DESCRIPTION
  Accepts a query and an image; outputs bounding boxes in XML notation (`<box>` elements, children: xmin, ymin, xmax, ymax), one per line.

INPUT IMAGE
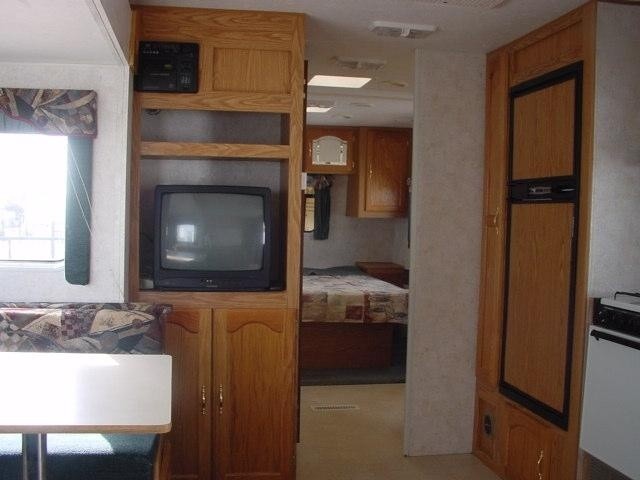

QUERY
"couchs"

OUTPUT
<box><xmin>0</xmin><ymin>304</ymin><xmax>173</xmax><ymax>479</ymax></box>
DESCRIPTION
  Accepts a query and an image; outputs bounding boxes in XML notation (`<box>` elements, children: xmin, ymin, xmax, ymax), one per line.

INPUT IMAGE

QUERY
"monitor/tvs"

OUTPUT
<box><xmin>153</xmin><ymin>185</ymin><xmax>272</xmax><ymax>292</ymax></box>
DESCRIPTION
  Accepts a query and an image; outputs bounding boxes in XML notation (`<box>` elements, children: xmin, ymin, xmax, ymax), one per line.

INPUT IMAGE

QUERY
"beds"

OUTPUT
<box><xmin>299</xmin><ymin>268</ymin><xmax>410</xmax><ymax>370</ymax></box>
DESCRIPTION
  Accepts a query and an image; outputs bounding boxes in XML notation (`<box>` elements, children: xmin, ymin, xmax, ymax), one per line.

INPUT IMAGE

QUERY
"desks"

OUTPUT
<box><xmin>0</xmin><ymin>351</ymin><xmax>173</xmax><ymax>479</ymax></box>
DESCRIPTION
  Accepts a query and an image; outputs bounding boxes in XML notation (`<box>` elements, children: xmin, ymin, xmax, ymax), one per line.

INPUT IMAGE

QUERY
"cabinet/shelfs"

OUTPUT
<box><xmin>344</xmin><ymin>128</ymin><xmax>415</xmax><ymax>220</ymax></box>
<box><xmin>303</xmin><ymin>123</ymin><xmax>357</xmax><ymax>173</ymax></box>
<box><xmin>469</xmin><ymin>0</ymin><xmax>598</xmax><ymax>480</ymax></box>
<box><xmin>129</xmin><ymin>7</ymin><xmax>306</xmax><ymax>480</ymax></box>
